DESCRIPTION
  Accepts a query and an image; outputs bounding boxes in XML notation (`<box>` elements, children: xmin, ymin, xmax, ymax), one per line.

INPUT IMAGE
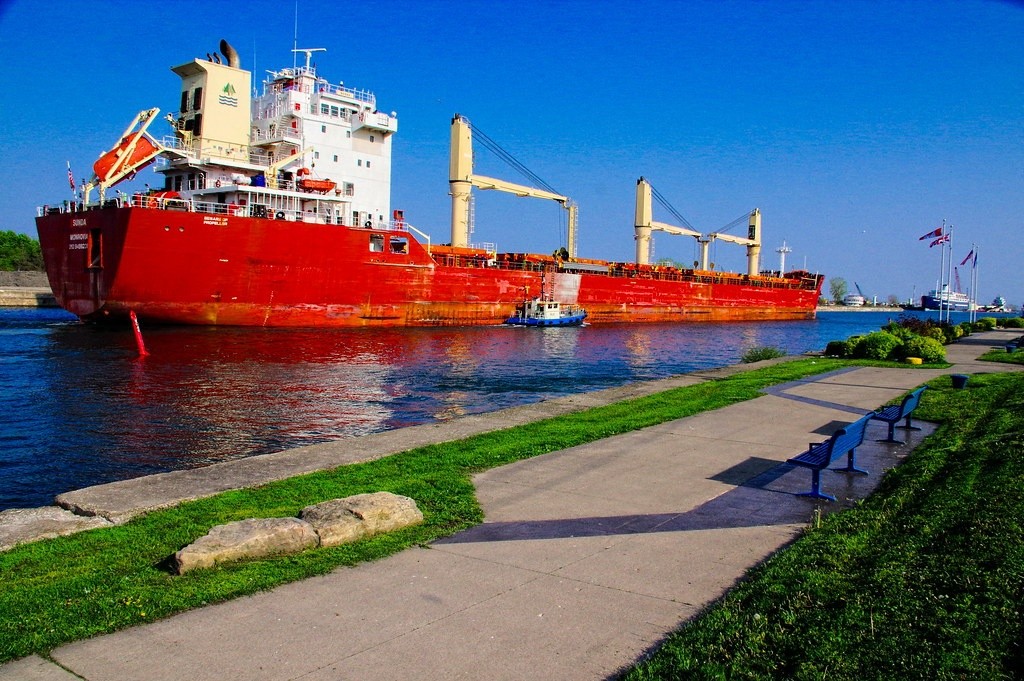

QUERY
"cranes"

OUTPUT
<box><xmin>953</xmin><ymin>267</ymin><xmax>961</xmax><ymax>293</ymax></box>
<box><xmin>854</xmin><ymin>281</ymin><xmax>861</xmax><ymax>296</ymax></box>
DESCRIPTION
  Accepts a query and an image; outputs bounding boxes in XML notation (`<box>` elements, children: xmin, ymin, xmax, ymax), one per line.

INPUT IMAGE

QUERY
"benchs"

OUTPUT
<box><xmin>869</xmin><ymin>385</ymin><xmax>926</xmax><ymax>446</ymax></box>
<box><xmin>789</xmin><ymin>414</ymin><xmax>873</xmax><ymax>501</ymax></box>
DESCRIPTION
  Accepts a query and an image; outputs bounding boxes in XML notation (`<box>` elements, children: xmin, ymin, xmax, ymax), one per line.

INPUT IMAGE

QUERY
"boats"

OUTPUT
<box><xmin>300</xmin><ymin>178</ymin><xmax>336</xmax><ymax>191</ymax></box>
<box><xmin>502</xmin><ymin>273</ymin><xmax>588</xmax><ymax>328</ymax></box>
<box><xmin>921</xmin><ymin>280</ymin><xmax>969</xmax><ymax>311</ymax></box>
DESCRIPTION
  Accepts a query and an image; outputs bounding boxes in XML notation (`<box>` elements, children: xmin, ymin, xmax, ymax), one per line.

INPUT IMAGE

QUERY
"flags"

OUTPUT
<box><xmin>972</xmin><ymin>251</ymin><xmax>977</xmax><ymax>267</ymax></box>
<box><xmin>68</xmin><ymin>158</ymin><xmax>75</xmax><ymax>189</ymax></box>
<box><xmin>929</xmin><ymin>233</ymin><xmax>950</xmax><ymax>248</ymax></box>
<box><xmin>960</xmin><ymin>249</ymin><xmax>973</xmax><ymax>265</ymax></box>
<box><xmin>919</xmin><ymin>227</ymin><xmax>943</xmax><ymax>240</ymax></box>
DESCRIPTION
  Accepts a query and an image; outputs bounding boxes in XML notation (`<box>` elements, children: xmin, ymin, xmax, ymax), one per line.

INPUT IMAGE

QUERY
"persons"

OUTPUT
<box><xmin>228</xmin><ymin>200</ymin><xmax>240</xmax><ymax>215</ymax></box>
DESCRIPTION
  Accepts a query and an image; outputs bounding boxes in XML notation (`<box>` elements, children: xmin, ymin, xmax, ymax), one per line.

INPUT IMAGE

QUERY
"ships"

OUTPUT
<box><xmin>34</xmin><ymin>0</ymin><xmax>826</xmax><ymax>356</ymax></box>
